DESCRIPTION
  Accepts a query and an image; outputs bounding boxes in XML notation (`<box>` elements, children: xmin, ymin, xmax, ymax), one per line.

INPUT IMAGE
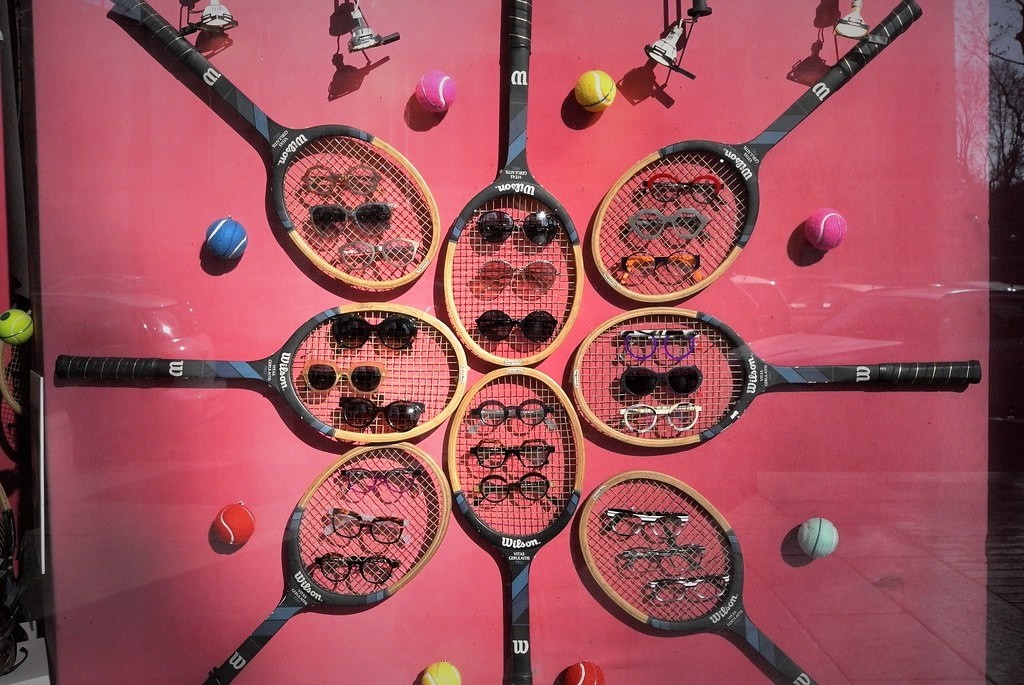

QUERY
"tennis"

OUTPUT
<box><xmin>802</xmin><ymin>206</ymin><xmax>848</xmax><ymax>251</ymax></box>
<box><xmin>0</xmin><ymin>309</ymin><xmax>35</xmax><ymax>346</ymax></box>
<box><xmin>214</xmin><ymin>502</ymin><xmax>256</xmax><ymax>546</ymax></box>
<box><xmin>206</xmin><ymin>218</ymin><xmax>248</xmax><ymax>261</ymax></box>
<box><xmin>415</xmin><ymin>70</ymin><xmax>456</xmax><ymax>113</ymax></box>
<box><xmin>574</xmin><ymin>68</ymin><xmax>618</xmax><ymax>112</ymax></box>
<box><xmin>423</xmin><ymin>661</ymin><xmax>463</xmax><ymax>685</ymax></box>
<box><xmin>798</xmin><ymin>516</ymin><xmax>840</xmax><ymax>558</ymax></box>
<box><xmin>563</xmin><ymin>659</ymin><xmax>606</xmax><ymax>685</ymax></box>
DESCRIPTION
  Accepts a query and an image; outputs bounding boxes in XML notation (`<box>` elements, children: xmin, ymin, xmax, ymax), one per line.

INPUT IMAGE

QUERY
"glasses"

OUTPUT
<box><xmin>315</xmin><ymin>552</ymin><xmax>402</xmax><ymax>584</ymax></box>
<box><xmin>471</xmin><ymin>400</ymin><xmax>556</xmax><ymax>432</ymax></box>
<box><xmin>642</xmin><ymin>171</ymin><xmax>726</xmax><ymax>203</ymax></box>
<box><xmin>469</xmin><ymin>436</ymin><xmax>556</xmax><ymax>469</ymax></box>
<box><xmin>302</xmin><ymin>162</ymin><xmax>383</xmax><ymax>196</ymax></box>
<box><xmin>476</xmin><ymin>211</ymin><xmax>561</xmax><ymax>245</ymax></box>
<box><xmin>302</xmin><ymin>360</ymin><xmax>387</xmax><ymax>394</ymax></box>
<box><xmin>327</xmin><ymin>508</ymin><xmax>408</xmax><ymax>544</ymax></box>
<box><xmin>622</xmin><ymin>544</ymin><xmax>706</xmax><ymax>573</ymax></box>
<box><xmin>335</xmin><ymin>238</ymin><xmax>426</xmax><ymax>270</ymax></box>
<box><xmin>474</xmin><ymin>472</ymin><xmax>553</xmax><ymax>512</ymax></box>
<box><xmin>647</xmin><ymin>573</ymin><xmax>730</xmax><ymax>605</ymax></box>
<box><xmin>340</xmin><ymin>469</ymin><xmax>421</xmax><ymax>495</ymax></box>
<box><xmin>308</xmin><ymin>203</ymin><xmax>397</xmax><ymax>236</ymax></box>
<box><xmin>621</xmin><ymin>208</ymin><xmax>712</xmax><ymax>243</ymax></box>
<box><xmin>331</xmin><ymin>316</ymin><xmax>419</xmax><ymax>353</ymax></box>
<box><xmin>475</xmin><ymin>311</ymin><xmax>559</xmax><ymax>343</ymax></box>
<box><xmin>620</xmin><ymin>250</ymin><xmax>702</xmax><ymax>287</ymax></box>
<box><xmin>604</xmin><ymin>507</ymin><xmax>689</xmax><ymax>546</ymax></box>
<box><xmin>617</xmin><ymin>329</ymin><xmax>701</xmax><ymax>364</ymax></box>
<box><xmin>476</xmin><ymin>259</ymin><xmax>560</xmax><ymax>293</ymax></box>
<box><xmin>616</xmin><ymin>364</ymin><xmax>704</xmax><ymax>403</ymax></box>
<box><xmin>619</xmin><ymin>404</ymin><xmax>702</xmax><ymax>433</ymax></box>
<box><xmin>338</xmin><ymin>398</ymin><xmax>426</xmax><ymax>432</ymax></box>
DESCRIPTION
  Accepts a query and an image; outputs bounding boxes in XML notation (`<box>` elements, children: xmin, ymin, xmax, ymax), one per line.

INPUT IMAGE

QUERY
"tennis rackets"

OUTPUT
<box><xmin>571</xmin><ymin>307</ymin><xmax>983</xmax><ymax>448</ymax></box>
<box><xmin>106</xmin><ymin>0</ymin><xmax>443</xmax><ymax>292</ymax></box>
<box><xmin>51</xmin><ymin>301</ymin><xmax>468</xmax><ymax>444</ymax></box>
<box><xmin>589</xmin><ymin>0</ymin><xmax>925</xmax><ymax>303</ymax></box>
<box><xmin>576</xmin><ymin>469</ymin><xmax>821</xmax><ymax>685</ymax></box>
<box><xmin>200</xmin><ymin>443</ymin><xmax>451</xmax><ymax>684</ymax></box>
<box><xmin>447</xmin><ymin>366</ymin><xmax>589</xmax><ymax>685</ymax></box>
<box><xmin>443</xmin><ymin>1</ymin><xmax>586</xmax><ymax>368</ymax></box>
